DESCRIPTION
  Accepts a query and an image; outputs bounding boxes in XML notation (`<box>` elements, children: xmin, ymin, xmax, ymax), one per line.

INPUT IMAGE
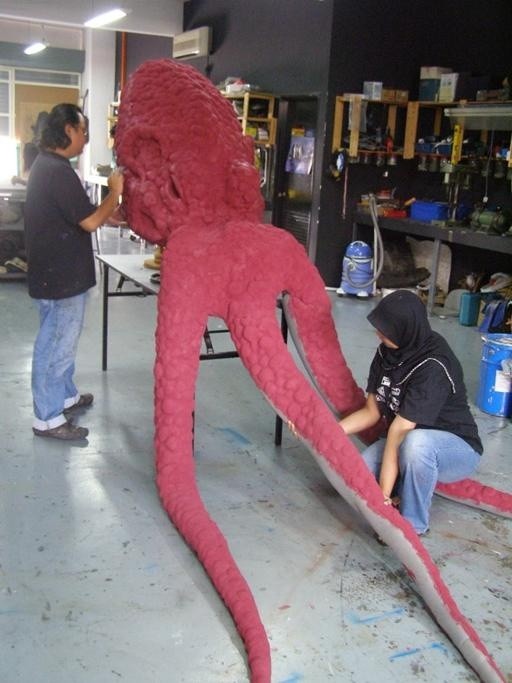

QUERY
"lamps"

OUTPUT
<box><xmin>23</xmin><ymin>22</ymin><xmax>49</xmax><ymax>56</ymax></box>
<box><xmin>83</xmin><ymin>0</ymin><xmax>127</xmax><ymax>29</ymax></box>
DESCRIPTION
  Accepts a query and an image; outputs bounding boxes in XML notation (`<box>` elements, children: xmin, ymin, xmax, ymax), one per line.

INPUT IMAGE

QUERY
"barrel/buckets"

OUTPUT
<box><xmin>459</xmin><ymin>292</ymin><xmax>478</xmax><ymax>327</ymax></box>
<box><xmin>478</xmin><ymin>334</ymin><xmax>512</xmax><ymax>418</ymax></box>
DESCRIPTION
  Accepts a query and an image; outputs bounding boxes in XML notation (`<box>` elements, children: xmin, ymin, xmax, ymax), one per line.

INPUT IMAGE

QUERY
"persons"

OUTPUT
<box><xmin>11</xmin><ymin>141</ymin><xmax>39</xmax><ymax>187</ymax></box>
<box><xmin>287</xmin><ymin>289</ymin><xmax>486</xmax><ymax>548</ymax></box>
<box><xmin>22</xmin><ymin>102</ymin><xmax>125</xmax><ymax>442</ymax></box>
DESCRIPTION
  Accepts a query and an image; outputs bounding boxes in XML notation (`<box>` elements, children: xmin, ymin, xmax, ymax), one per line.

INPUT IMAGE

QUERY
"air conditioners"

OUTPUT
<box><xmin>172</xmin><ymin>25</ymin><xmax>212</xmax><ymax>62</ymax></box>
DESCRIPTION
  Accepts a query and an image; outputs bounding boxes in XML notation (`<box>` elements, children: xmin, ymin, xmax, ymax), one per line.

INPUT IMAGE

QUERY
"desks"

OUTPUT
<box><xmin>347</xmin><ymin>210</ymin><xmax>512</xmax><ymax>319</ymax></box>
<box><xmin>93</xmin><ymin>252</ymin><xmax>289</xmax><ymax>447</ymax></box>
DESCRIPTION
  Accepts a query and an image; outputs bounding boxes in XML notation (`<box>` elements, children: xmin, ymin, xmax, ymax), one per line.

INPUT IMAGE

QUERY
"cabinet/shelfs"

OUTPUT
<box><xmin>223</xmin><ymin>91</ymin><xmax>277</xmax><ymax>147</ymax></box>
<box><xmin>332</xmin><ymin>94</ymin><xmax>511</xmax><ymax>169</ymax></box>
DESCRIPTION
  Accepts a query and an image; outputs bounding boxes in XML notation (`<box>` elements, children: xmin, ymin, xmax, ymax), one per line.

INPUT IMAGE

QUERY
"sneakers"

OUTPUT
<box><xmin>63</xmin><ymin>392</ymin><xmax>93</xmax><ymax>414</ymax></box>
<box><xmin>32</xmin><ymin>421</ymin><xmax>89</xmax><ymax>440</ymax></box>
